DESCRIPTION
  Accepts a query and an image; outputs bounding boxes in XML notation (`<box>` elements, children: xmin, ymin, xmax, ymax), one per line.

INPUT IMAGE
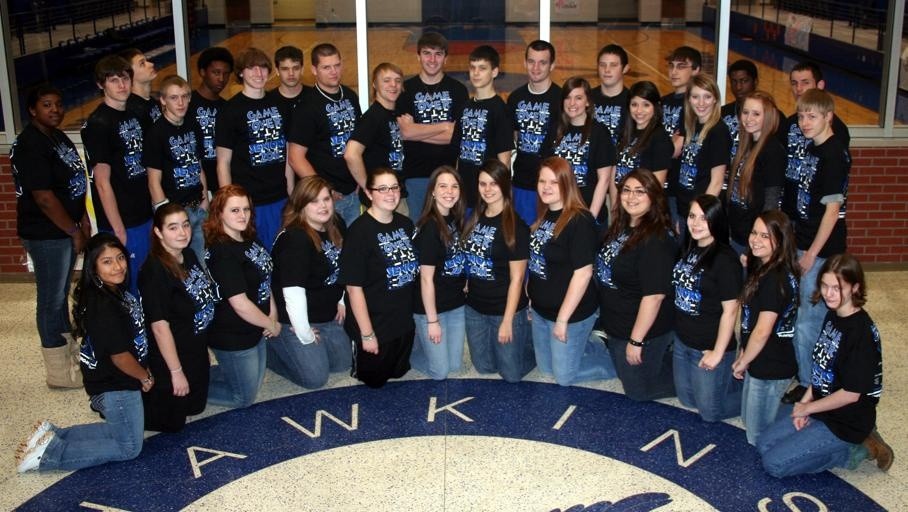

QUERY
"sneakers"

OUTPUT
<box><xmin>15</xmin><ymin>419</ymin><xmax>53</xmax><ymax>465</ymax></box>
<box><xmin>18</xmin><ymin>431</ymin><xmax>56</xmax><ymax>475</ymax></box>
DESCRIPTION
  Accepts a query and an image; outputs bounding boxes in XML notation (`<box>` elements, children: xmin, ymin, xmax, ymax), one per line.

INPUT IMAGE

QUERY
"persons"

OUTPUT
<box><xmin>81</xmin><ymin>32</ymin><xmax>854</xmax><ymax>451</ymax></box>
<box><xmin>755</xmin><ymin>254</ymin><xmax>895</xmax><ymax>479</ymax></box>
<box><xmin>13</xmin><ymin>230</ymin><xmax>156</xmax><ymax>474</ymax></box>
<box><xmin>11</xmin><ymin>81</ymin><xmax>93</xmax><ymax>391</ymax></box>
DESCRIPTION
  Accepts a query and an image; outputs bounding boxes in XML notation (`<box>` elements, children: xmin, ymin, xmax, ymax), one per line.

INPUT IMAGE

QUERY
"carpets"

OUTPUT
<box><xmin>406</xmin><ymin>26</ymin><xmax>524</xmax><ymax>43</ymax></box>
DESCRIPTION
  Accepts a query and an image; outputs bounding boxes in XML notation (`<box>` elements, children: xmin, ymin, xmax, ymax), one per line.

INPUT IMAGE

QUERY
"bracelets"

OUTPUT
<box><xmin>68</xmin><ymin>221</ymin><xmax>80</xmax><ymax>236</ymax></box>
<box><xmin>140</xmin><ymin>371</ymin><xmax>152</xmax><ymax>386</ymax></box>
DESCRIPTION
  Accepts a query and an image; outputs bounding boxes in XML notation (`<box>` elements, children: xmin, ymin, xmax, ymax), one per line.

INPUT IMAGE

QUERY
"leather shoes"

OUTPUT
<box><xmin>780</xmin><ymin>385</ymin><xmax>808</xmax><ymax>405</ymax></box>
<box><xmin>863</xmin><ymin>430</ymin><xmax>894</xmax><ymax>471</ymax></box>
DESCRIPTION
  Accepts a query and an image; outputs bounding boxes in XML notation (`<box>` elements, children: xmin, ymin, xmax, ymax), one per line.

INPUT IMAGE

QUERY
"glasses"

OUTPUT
<box><xmin>369</xmin><ymin>184</ymin><xmax>401</xmax><ymax>194</ymax></box>
<box><xmin>620</xmin><ymin>187</ymin><xmax>648</xmax><ymax>197</ymax></box>
<box><xmin>665</xmin><ymin>62</ymin><xmax>692</xmax><ymax>69</ymax></box>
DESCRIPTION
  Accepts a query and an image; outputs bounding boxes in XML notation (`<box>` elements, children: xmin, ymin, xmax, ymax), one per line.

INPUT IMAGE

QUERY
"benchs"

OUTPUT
<box><xmin>143</xmin><ymin>44</ymin><xmax>175</xmax><ymax>64</ymax></box>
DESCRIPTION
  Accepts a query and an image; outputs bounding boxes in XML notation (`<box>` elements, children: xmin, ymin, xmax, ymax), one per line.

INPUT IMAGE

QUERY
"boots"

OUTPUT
<box><xmin>62</xmin><ymin>333</ymin><xmax>82</xmax><ymax>365</ymax></box>
<box><xmin>42</xmin><ymin>346</ymin><xmax>84</xmax><ymax>391</ymax></box>
